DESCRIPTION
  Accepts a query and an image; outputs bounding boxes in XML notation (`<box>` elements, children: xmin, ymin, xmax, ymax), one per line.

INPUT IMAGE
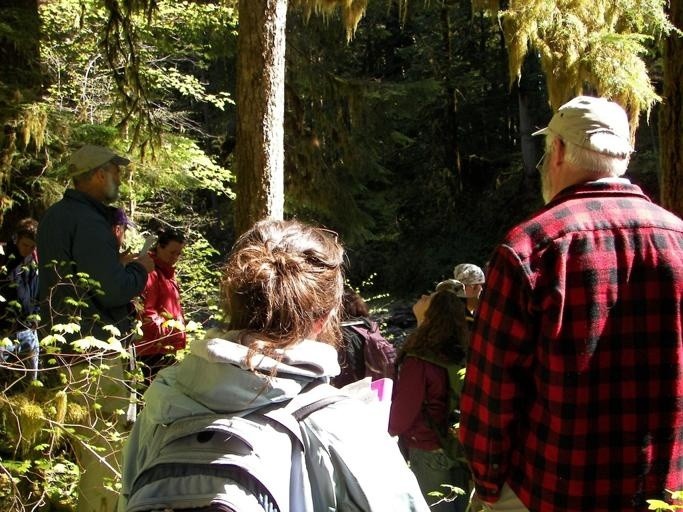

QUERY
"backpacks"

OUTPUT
<box><xmin>125</xmin><ymin>387</ymin><xmax>349</xmax><ymax>512</ymax></box>
<box><xmin>350</xmin><ymin>322</ymin><xmax>398</xmax><ymax>382</ymax></box>
<box><xmin>401</xmin><ymin>351</ymin><xmax>468</xmax><ymax>461</ymax></box>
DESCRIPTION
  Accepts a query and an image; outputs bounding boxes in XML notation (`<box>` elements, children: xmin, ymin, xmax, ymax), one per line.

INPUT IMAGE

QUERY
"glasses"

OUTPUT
<box><xmin>536</xmin><ymin>151</ymin><xmax>550</xmax><ymax>173</ymax></box>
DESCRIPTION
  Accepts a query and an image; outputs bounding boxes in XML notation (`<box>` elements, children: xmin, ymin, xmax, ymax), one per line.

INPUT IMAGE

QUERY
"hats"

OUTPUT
<box><xmin>69</xmin><ymin>144</ymin><xmax>131</xmax><ymax>177</ymax></box>
<box><xmin>108</xmin><ymin>207</ymin><xmax>138</xmax><ymax>228</ymax></box>
<box><xmin>435</xmin><ymin>263</ymin><xmax>486</xmax><ymax>298</ymax></box>
<box><xmin>531</xmin><ymin>95</ymin><xmax>630</xmax><ymax>156</ymax></box>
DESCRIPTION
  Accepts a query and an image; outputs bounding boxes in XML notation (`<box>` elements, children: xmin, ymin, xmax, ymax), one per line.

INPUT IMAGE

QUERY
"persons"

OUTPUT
<box><xmin>457</xmin><ymin>96</ymin><xmax>683</xmax><ymax>511</ymax></box>
<box><xmin>120</xmin><ymin>219</ymin><xmax>488</xmax><ymax>512</ymax></box>
<box><xmin>2</xmin><ymin>143</ymin><xmax>187</xmax><ymax>396</ymax></box>
